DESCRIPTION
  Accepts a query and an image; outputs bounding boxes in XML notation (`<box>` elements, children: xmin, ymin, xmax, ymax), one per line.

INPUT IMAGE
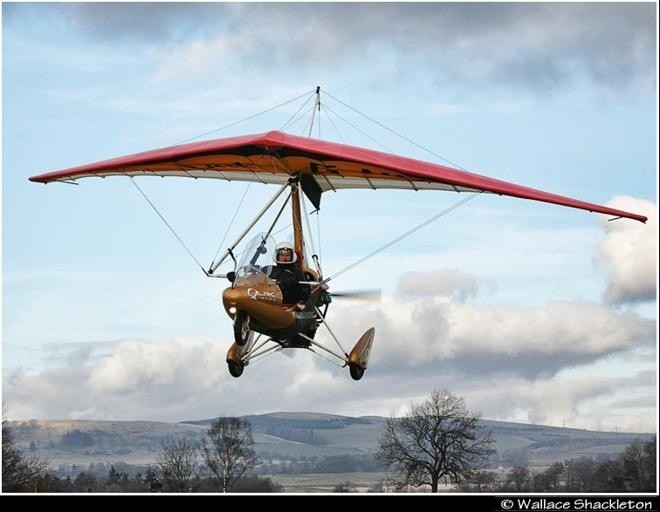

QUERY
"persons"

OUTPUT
<box><xmin>260</xmin><ymin>240</ymin><xmax>313</xmax><ymax>316</ymax></box>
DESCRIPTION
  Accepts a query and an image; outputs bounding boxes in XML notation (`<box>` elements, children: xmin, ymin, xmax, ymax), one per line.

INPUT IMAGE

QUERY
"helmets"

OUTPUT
<box><xmin>273</xmin><ymin>242</ymin><xmax>297</xmax><ymax>264</ymax></box>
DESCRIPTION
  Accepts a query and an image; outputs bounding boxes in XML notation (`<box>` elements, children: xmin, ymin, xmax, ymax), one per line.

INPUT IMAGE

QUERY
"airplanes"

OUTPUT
<box><xmin>26</xmin><ymin>88</ymin><xmax>649</xmax><ymax>381</ymax></box>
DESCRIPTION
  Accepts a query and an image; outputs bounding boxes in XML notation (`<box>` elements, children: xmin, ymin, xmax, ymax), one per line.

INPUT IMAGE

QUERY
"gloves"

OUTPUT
<box><xmin>292</xmin><ymin>300</ymin><xmax>305</xmax><ymax>312</ymax></box>
